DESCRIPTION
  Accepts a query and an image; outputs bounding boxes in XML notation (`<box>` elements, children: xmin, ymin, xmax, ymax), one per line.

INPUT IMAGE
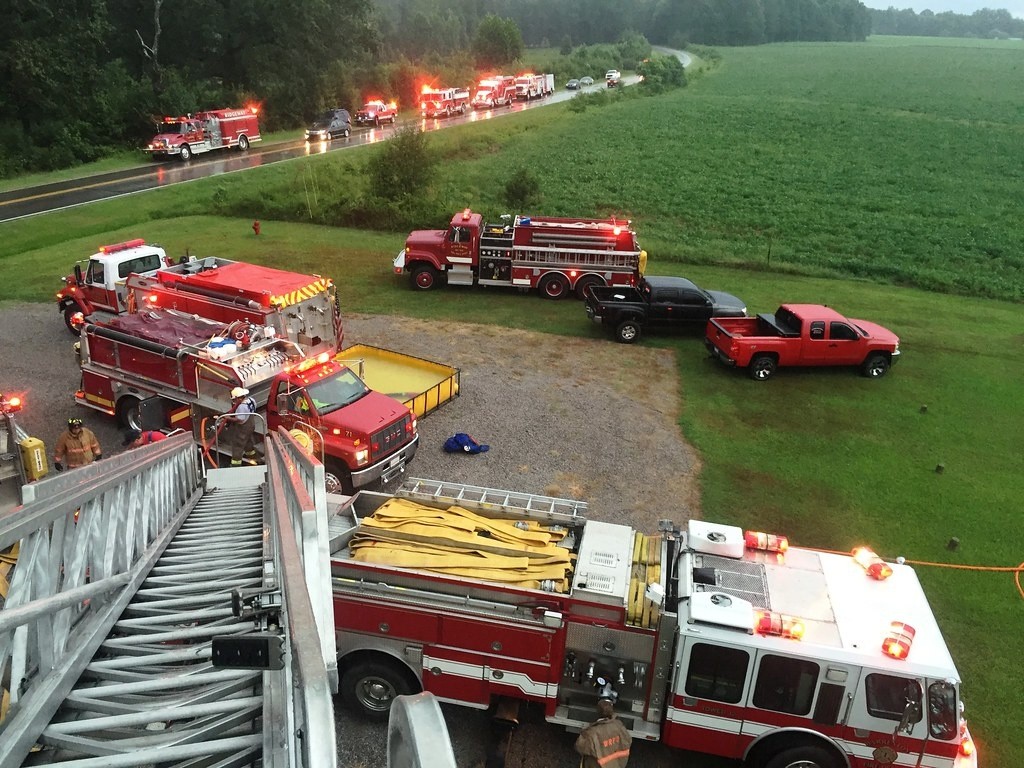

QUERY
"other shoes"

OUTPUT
<box><xmin>242</xmin><ymin>453</ymin><xmax>256</xmax><ymax>458</ymax></box>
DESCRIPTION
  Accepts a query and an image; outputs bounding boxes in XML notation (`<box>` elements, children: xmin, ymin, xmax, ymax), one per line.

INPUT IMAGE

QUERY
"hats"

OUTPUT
<box><xmin>120</xmin><ymin>429</ymin><xmax>140</xmax><ymax>445</ymax></box>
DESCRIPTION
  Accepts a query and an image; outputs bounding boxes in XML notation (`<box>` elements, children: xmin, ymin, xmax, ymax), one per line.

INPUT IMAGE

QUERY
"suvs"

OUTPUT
<box><xmin>313</xmin><ymin>108</ymin><xmax>352</xmax><ymax>125</ymax></box>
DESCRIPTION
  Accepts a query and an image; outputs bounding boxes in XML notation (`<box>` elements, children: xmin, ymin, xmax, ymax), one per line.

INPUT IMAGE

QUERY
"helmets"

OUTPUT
<box><xmin>67</xmin><ymin>417</ymin><xmax>83</xmax><ymax>427</ymax></box>
<box><xmin>229</xmin><ymin>387</ymin><xmax>249</xmax><ymax>399</ymax></box>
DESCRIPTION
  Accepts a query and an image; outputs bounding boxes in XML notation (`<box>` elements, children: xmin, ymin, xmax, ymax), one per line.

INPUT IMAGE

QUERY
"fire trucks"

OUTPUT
<box><xmin>57</xmin><ymin>239</ymin><xmax>344</xmax><ymax>356</ymax></box>
<box><xmin>392</xmin><ymin>207</ymin><xmax>647</xmax><ymax>301</ymax></box>
<box><xmin>471</xmin><ymin>75</ymin><xmax>518</xmax><ymax>110</ymax></box>
<box><xmin>514</xmin><ymin>72</ymin><xmax>556</xmax><ymax>100</ymax></box>
<box><xmin>71</xmin><ymin>296</ymin><xmax>420</xmax><ymax>495</ymax></box>
<box><xmin>323</xmin><ymin>477</ymin><xmax>976</xmax><ymax>768</ymax></box>
<box><xmin>421</xmin><ymin>87</ymin><xmax>470</xmax><ymax>119</ymax></box>
<box><xmin>141</xmin><ymin>108</ymin><xmax>262</xmax><ymax>162</ymax></box>
<box><xmin>354</xmin><ymin>100</ymin><xmax>399</xmax><ymax>126</ymax></box>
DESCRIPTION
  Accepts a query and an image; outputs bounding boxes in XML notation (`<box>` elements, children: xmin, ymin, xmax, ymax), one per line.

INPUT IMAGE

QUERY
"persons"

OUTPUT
<box><xmin>575</xmin><ymin>700</ymin><xmax>632</xmax><ymax>768</ymax></box>
<box><xmin>121</xmin><ymin>428</ymin><xmax>166</xmax><ymax>449</ymax></box>
<box><xmin>53</xmin><ymin>418</ymin><xmax>102</xmax><ymax>472</ymax></box>
<box><xmin>294</xmin><ymin>388</ymin><xmax>330</xmax><ymax>415</ymax></box>
<box><xmin>224</xmin><ymin>386</ymin><xmax>259</xmax><ymax>467</ymax></box>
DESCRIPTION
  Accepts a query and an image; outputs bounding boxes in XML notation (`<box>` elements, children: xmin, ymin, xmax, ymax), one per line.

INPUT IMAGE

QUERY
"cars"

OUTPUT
<box><xmin>608</xmin><ymin>78</ymin><xmax>619</xmax><ymax>88</ymax></box>
<box><xmin>580</xmin><ymin>77</ymin><xmax>593</xmax><ymax>86</ymax></box>
<box><xmin>304</xmin><ymin>119</ymin><xmax>353</xmax><ymax>142</ymax></box>
<box><xmin>565</xmin><ymin>79</ymin><xmax>580</xmax><ymax>90</ymax></box>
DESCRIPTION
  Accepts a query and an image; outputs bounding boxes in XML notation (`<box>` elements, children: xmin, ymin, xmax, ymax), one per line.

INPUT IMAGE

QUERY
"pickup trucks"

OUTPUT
<box><xmin>704</xmin><ymin>303</ymin><xmax>900</xmax><ymax>382</ymax></box>
<box><xmin>586</xmin><ymin>276</ymin><xmax>748</xmax><ymax>344</ymax></box>
<box><xmin>605</xmin><ymin>70</ymin><xmax>620</xmax><ymax>81</ymax></box>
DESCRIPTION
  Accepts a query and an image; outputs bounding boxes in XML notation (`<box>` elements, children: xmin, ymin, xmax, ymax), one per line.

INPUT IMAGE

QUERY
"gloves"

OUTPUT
<box><xmin>54</xmin><ymin>463</ymin><xmax>63</xmax><ymax>471</ymax></box>
<box><xmin>94</xmin><ymin>454</ymin><xmax>101</xmax><ymax>461</ymax></box>
